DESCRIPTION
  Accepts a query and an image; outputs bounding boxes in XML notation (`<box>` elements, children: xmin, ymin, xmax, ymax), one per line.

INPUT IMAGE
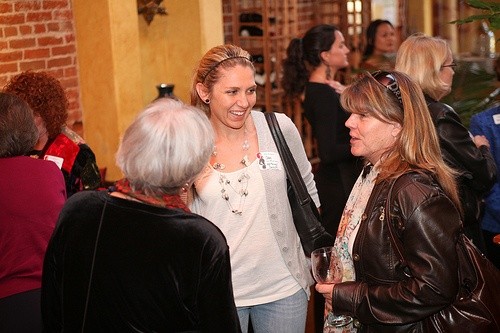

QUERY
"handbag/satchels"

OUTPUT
<box><xmin>384</xmin><ymin>167</ymin><xmax>500</xmax><ymax>333</ymax></box>
<box><xmin>261</xmin><ymin>110</ymin><xmax>335</xmax><ymax>259</ymax></box>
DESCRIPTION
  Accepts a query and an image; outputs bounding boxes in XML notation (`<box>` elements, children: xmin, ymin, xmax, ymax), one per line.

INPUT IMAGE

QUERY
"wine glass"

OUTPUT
<box><xmin>311</xmin><ymin>246</ymin><xmax>353</xmax><ymax>327</ymax></box>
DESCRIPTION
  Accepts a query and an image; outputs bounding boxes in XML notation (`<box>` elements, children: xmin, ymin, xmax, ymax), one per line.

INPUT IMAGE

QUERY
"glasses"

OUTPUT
<box><xmin>371</xmin><ymin>70</ymin><xmax>404</xmax><ymax>110</ymax></box>
<box><xmin>441</xmin><ymin>61</ymin><xmax>458</xmax><ymax>74</ymax></box>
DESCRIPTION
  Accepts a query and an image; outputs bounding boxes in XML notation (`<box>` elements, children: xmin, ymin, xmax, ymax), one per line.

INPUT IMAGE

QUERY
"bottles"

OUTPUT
<box><xmin>153</xmin><ymin>82</ymin><xmax>179</xmax><ymax>101</ymax></box>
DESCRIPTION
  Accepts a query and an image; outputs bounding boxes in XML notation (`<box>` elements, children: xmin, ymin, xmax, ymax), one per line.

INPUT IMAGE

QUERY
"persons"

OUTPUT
<box><xmin>394</xmin><ymin>32</ymin><xmax>496</xmax><ymax>243</ymax></box>
<box><xmin>468</xmin><ymin>56</ymin><xmax>500</xmax><ymax>263</ymax></box>
<box><xmin>0</xmin><ymin>92</ymin><xmax>67</xmax><ymax>333</ymax></box>
<box><xmin>281</xmin><ymin>23</ymin><xmax>364</xmax><ymax>240</ymax></box>
<box><xmin>316</xmin><ymin>70</ymin><xmax>463</xmax><ymax>333</ymax></box>
<box><xmin>356</xmin><ymin>19</ymin><xmax>398</xmax><ymax>79</ymax></box>
<box><xmin>3</xmin><ymin>69</ymin><xmax>101</xmax><ymax>197</ymax></box>
<box><xmin>182</xmin><ymin>44</ymin><xmax>321</xmax><ymax>333</ymax></box>
<box><xmin>42</xmin><ymin>98</ymin><xmax>243</xmax><ymax>333</ymax></box>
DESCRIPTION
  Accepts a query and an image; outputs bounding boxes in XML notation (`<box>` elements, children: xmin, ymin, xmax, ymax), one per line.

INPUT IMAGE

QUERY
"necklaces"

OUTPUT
<box><xmin>210</xmin><ymin>122</ymin><xmax>249</xmax><ymax>216</ymax></box>
